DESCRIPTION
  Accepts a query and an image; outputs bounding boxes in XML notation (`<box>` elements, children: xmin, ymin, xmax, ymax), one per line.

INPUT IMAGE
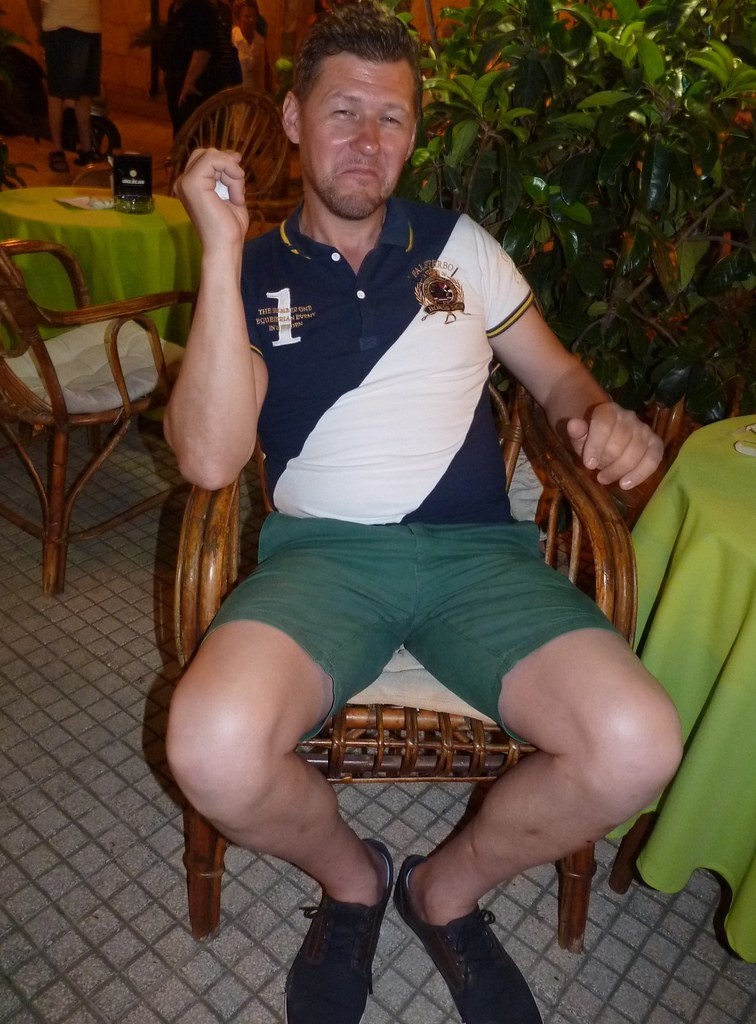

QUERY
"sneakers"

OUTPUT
<box><xmin>280</xmin><ymin>841</ymin><xmax>394</xmax><ymax>1024</ymax></box>
<box><xmin>396</xmin><ymin>855</ymin><xmax>545</xmax><ymax>1024</ymax></box>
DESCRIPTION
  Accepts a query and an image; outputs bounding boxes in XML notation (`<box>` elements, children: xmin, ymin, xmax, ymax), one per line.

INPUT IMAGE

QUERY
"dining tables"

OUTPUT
<box><xmin>629</xmin><ymin>412</ymin><xmax>756</xmax><ymax>966</ymax></box>
<box><xmin>0</xmin><ymin>186</ymin><xmax>204</xmax><ymax>360</ymax></box>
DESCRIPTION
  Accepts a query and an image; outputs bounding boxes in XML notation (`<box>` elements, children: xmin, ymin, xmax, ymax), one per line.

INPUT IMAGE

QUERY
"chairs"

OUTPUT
<box><xmin>70</xmin><ymin>87</ymin><xmax>290</xmax><ymax>202</ymax></box>
<box><xmin>170</xmin><ymin>365</ymin><xmax>643</xmax><ymax>955</ymax></box>
<box><xmin>244</xmin><ymin>182</ymin><xmax>313</xmax><ymax>245</ymax></box>
<box><xmin>0</xmin><ymin>238</ymin><xmax>201</xmax><ymax>601</ymax></box>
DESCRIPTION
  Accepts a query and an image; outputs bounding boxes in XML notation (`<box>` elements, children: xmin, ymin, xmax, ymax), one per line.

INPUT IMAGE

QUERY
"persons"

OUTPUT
<box><xmin>164</xmin><ymin>1</ymin><xmax>685</xmax><ymax>1024</ymax></box>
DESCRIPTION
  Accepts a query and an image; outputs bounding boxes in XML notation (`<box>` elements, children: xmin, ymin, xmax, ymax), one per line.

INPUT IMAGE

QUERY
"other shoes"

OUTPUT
<box><xmin>45</xmin><ymin>145</ymin><xmax>104</xmax><ymax>173</ymax></box>
